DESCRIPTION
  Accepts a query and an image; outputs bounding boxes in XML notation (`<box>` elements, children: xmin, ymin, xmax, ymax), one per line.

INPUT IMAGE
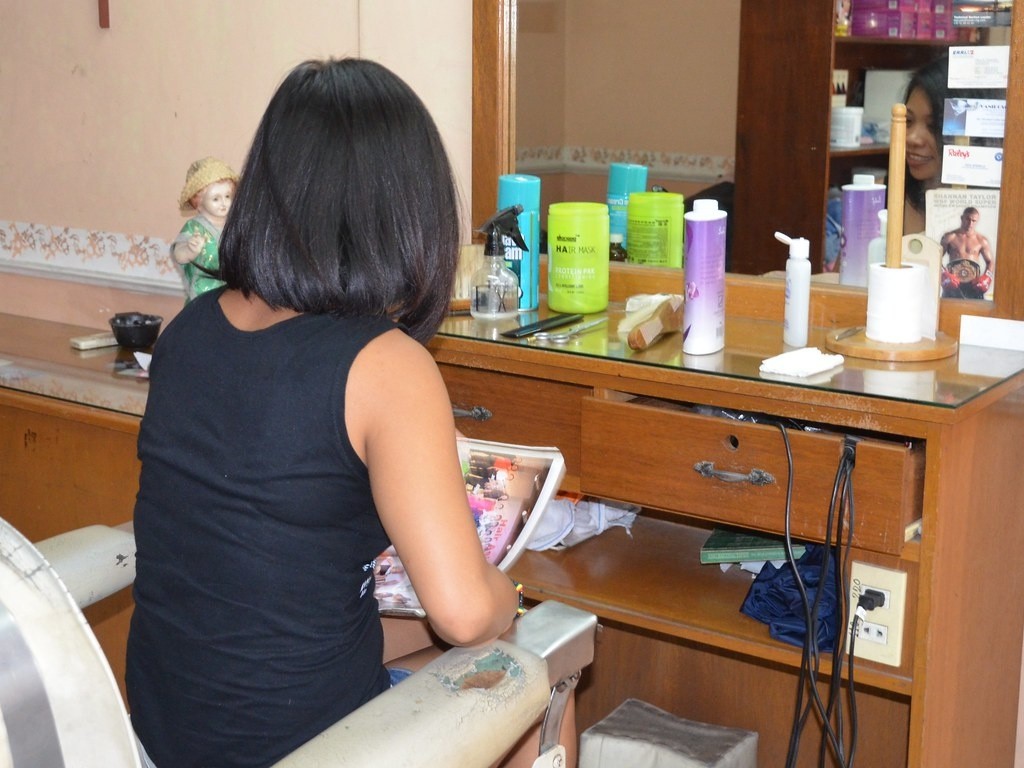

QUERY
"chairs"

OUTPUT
<box><xmin>1</xmin><ymin>519</ymin><xmax>597</xmax><ymax>767</ymax></box>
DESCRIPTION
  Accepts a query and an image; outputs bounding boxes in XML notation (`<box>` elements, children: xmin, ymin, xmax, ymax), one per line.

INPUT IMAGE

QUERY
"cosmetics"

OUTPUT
<box><xmin>774</xmin><ymin>230</ymin><xmax>812</xmax><ymax>347</ymax></box>
<box><xmin>841</xmin><ymin>173</ymin><xmax>886</xmax><ymax>285</ymax></box>
<box><xmin>547</xmin><ymin>202</ymin><xmax>610</xmax><ymax>313</ymax></box>
<box><xmin>497</xmin><ymin>173</ymin><xmax>539</xmax><ymax>312</ymax></box>
<box><xmin>685</xmin><ymin>200</ymin><xmax>727</xmax><ymax>355</ymax></box>
<box><xmin>610</xmin><ymin>163</ymin><xmax>684</xmax><ymax>269</ymax></box>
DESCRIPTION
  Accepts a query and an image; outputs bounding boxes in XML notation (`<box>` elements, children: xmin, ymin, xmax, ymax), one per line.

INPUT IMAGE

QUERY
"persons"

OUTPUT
<box><xmin>173</xmin><ymin>157</ymin><xmax>238</xmax><ymax>304</ymax></box>
<box><xmin>940</xmin><ymin>206</ymin><xmax>994</xmax><ymax>300</ymax></box>
<box><xmin>902</xmin><ymin>54</ymin><xmax>988</xmax><ymax>237</ymax></box>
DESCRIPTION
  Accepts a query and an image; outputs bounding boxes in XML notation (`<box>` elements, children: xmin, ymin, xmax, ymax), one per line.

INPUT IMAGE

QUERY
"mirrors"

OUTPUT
<box><xmin>513</xmin><ymin>2</ymin><xmax>1012</xmax><ymax>300</ymax></box>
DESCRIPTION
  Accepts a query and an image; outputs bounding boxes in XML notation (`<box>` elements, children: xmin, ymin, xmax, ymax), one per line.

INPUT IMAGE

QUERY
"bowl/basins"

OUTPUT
<box><xmin>109</xmin><ymin>308</ymin><xmax>163</xmax><ymax>351</ymax></box>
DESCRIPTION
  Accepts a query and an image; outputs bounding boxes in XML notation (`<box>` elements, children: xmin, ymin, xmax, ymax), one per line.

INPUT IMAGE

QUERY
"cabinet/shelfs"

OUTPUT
<box><xmin>732</xmin><ymin>0</ymin><xmax>986</xmax><ymax>275</ymax></box>
<box><xmin>2</xmin><ymin>313</ymin><xmax>157</xmax><ymax>715</ymax></box>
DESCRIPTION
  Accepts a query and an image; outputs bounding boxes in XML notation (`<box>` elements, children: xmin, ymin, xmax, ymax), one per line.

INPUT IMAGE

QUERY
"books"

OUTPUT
<box><xmin>372</xmin><ymin>436</ymin><xmax>567</xmax><ymax>620</ymax></box>
<box><xmin>699</xmin><ymin>522</ymin><xmax>807</xmax><ymax>564</ymax></box>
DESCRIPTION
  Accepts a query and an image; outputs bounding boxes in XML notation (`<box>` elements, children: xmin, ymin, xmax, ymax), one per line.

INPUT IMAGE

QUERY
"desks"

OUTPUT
<box><xmin>426</xmin><ymin>299</ymin><xmax>1022</xmax><ymax>767</ymax></box>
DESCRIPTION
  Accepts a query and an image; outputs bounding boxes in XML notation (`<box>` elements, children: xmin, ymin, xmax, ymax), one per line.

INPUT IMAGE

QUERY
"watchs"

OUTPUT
<box><xmin>124</xmin><ymin>58</ymin><xmax>519</xmax><ymax>767</ymax></box>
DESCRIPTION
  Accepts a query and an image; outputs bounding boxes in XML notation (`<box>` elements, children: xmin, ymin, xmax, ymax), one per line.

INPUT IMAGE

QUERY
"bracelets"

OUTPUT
<box><xmin>512</xmin><ymin>581</ymin><xmax>523</xmax><ymax>618</ymax></box>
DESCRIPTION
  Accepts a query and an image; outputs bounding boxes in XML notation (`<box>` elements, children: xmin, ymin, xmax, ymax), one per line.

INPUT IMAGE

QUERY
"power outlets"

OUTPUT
<box><xmin>845</xmin><ymin>561</ymin><xmax>906</xmax><ymax>667</ymax></box>
